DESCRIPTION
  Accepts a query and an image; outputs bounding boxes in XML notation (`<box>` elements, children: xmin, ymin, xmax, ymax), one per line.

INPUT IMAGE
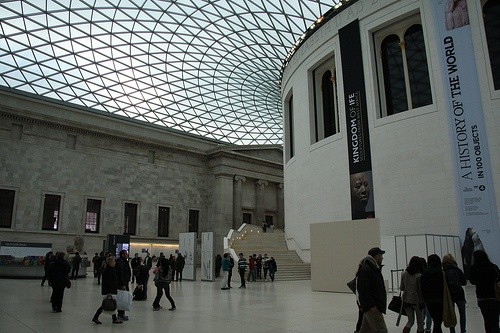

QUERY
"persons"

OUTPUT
<box><xmin>350</xmin><ymin>172</ymin><xmax>375</xmax><ymax>219</ymax></box>
<box><xmin>472</xmin><ymin>249</ymin><xmax>500</xmax><ymax>333</ymax></box>
<box><xmin>113</xmin><ymin>250</ymin><xmax>131</xmax><ymax>321</ymax></box>
<box><xmin>92</xmin><ymin>255</ymin><xmax>123</xmax><ymax>324</ymax></box>
<box><xmin>237</xmin><ymin>253</ymin><xmax>247</xmax><ymax>288</ymax></box>
<box><xmin>131</xmin><ymin>253</ymin><xmax>151</xmax><ymax>300</ymax></box>
<box><xmin>248</xmin><ymin>254</ymin><xmax>277</xmax><ymax>282</ymax></box>
<box><xmin>221</xmin><ymin>253</ymin><xmax>234</xmax><ymax>290</ymax></box>
<box><xmin>263</xmin><ymin>218</ymin><xmax>270</xmax><ymax>233</ymax></box>
<box><xmin>155</xmin><ymin>252</ymin><xmax>184</xmax><ymax>282</ymax></box>
<box><xmin>152</xmin><ymin>259</ymin><xmax>176</xmax><ymax>311</ymax></box>
<box><xmin>41</xmin><ymin>250</ymin><xmax>71</xmax><ymax>312</ymax></box>
<box><xmin>92</xmin><ymin>249</ymin><xmax>116</xmax><ymax>285</ymax></box>
<box><xmin>71</xmin><ymin>252</ymin><xmax>81</xmax><ymax>280</ymax></box>
<box><xmin>80</xmin><ymin>252</ymin><xmax>88</xmax><ymax>278</ymax></box>
<box><xmin>353</xmin><ymin>246</ymin><xmax>389</xmax><ymax>333</ymax></box>
<box><xmin>399</xmin><ymin>250</ymin><xmax>467</xmax><ymax>333</ymax></box>
<box><xmin>215</xmin><ymin>254</ymin><xmax>222</xmax><ymax>278</ymax></box>
<box><xmin>461</xmin><ymin>228</ymin><xmax>483</xmax><ymax>279</ymax></box>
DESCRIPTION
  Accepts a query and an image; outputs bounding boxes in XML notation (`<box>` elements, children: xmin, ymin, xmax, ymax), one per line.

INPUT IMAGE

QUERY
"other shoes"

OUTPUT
<box><xmin>112</xmin><ymin>319</ymin><xmax>122</xmax><ymax>324</ymax></box>
<box><xmin>169</xmin><ymin>306</ymin><xmax>177</xmax><ymax>310</ymax></box>
<box><xmin>92</xmin><ymin>318</ymin><xmax>102</xmax><ymax>324</ymax></box>
<box><xmin>119</xmin><ymin>315</ymin><xmax>128</xmax><ymax>319</ymax></box>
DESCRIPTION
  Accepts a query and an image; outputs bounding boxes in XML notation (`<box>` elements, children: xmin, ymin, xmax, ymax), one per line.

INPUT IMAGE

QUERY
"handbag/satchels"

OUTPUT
<box><xmin>389</xmin><ymin>295</ymin><xmax>407</xmax><ymax>316</ymax></box>
<box><xmin>102</xmin><ymin>294</ymin><xmax>117</xmax><ymax>311</ymax></box>
<box><xmin>443</xmin><ymin>300</ymin><xmax>458</xmax><ymax>327</ymax></box>
<box><xmin>66</xmin><ymin>278</ymin><xmax>71</xmax><ymax>288</ymax></box>
<box><xmin>113</xmin><ymin>289</ymin><xmax>132</xmax><ymax>311</ymax></box>
<box><xmin>132</xmin><ymin>286</ymin><xmax>148</xmax><ymax>300</ymax></box>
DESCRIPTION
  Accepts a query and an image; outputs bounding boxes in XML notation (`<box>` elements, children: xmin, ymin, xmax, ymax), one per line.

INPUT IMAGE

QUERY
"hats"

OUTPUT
<box><xmin>368</xmin><ymin>247</ymin><xmax>385</xmax><ymax>255</ymax></box>
